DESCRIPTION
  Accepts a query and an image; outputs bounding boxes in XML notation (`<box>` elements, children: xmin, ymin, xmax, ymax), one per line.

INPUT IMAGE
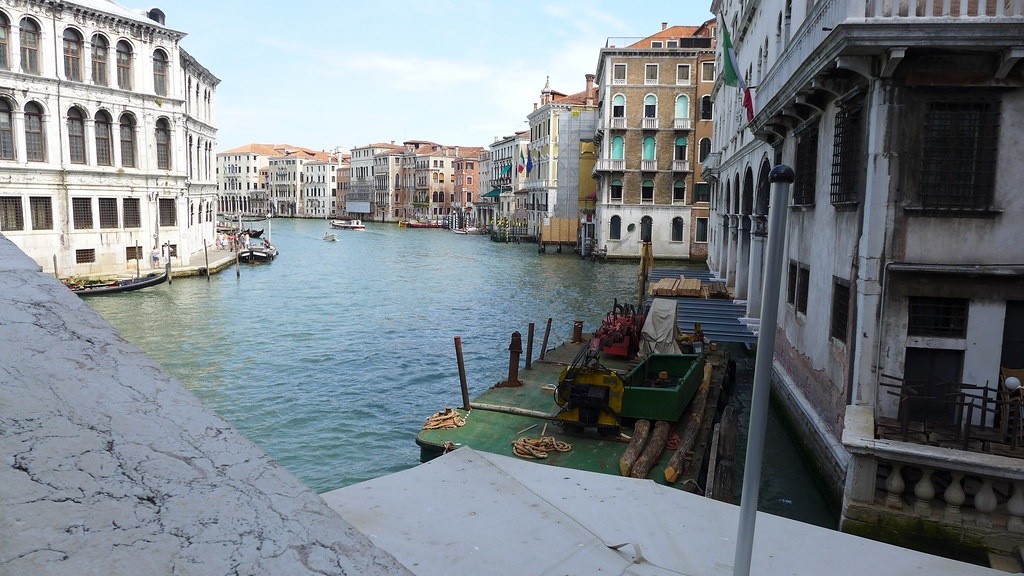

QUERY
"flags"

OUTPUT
<box><xmin>527</xmin><ymin>147</ymin><xmax>533</xmax><ymax>173</ymax></box>
<box><xmin>721</xmin><ymin>14</ymin><xmax>754</xmax><ymax>122</ymax></box>
<box><xmin>518</xmin><ymin>150</ymin><xmax>526</xmax><ymax>174</ymax></box>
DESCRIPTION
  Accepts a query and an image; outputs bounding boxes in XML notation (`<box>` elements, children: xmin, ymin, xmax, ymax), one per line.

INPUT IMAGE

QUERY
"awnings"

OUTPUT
<box><xmin>582</xmin><ymin>208</ymin><xmax>595</xmax><ymax>215</ymax></box>
<box><xmin>500</xmin><ymin>165</ymin><xmax>511</xmax><ymax>175</ymax></box>
<box><xmin>481</xmin><ymin>187</ymin><xmax>512</xmax><ymax>197</ymax></box>
<box><xmin>586</xmin><ymin>192</ymin><xmax>597</xmax><ymax>199</ymax></box>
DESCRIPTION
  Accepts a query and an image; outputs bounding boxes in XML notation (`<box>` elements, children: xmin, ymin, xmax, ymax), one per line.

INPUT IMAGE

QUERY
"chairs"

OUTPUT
<box><xmin>1000</xmin><ymin>367</ymin><xmax>1024</xmax><ymax>448</ymax></box>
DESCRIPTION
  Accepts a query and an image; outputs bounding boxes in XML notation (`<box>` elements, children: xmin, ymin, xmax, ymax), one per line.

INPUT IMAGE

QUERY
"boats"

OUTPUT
<box><xmin>322</xmin><ymin>231</ymin><xmax>338</xmax><ymax>242</ymax></box>
<box><xmin>224</xmin><ymin>215</ymin><xmax>267</xmax><ymax>222</ymax></box>
<box><xmin>400</xmin><ymin>219</ymin><xmax>450</xmax><ymax>227</ymax></box>
<box><xmin>60</xmin><ymin>271</ymin><xmax>168</xmax><ymax>296</ymax></box>
<box><xmin>236</xmin><ymin>243</ymin><xmax>277</xmax><ymax>262</ymax></box>
<box><xmin>329</xmin><ymin>219</ymin><xmax>366</xmax><ymax>230</ymax></box>
<box><xmin>217</xmin><ymin>222</ymin><xmax>265</xmax><ymax>238</ymax></box>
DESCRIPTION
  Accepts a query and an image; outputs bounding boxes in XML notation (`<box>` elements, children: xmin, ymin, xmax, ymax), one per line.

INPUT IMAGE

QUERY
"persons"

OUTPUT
<box><xmin>162</xmin><ymin>242</ymin><xmax>173</xmax><ymax>269</ymax></box>
<box><xmin>215</xmin><ymin>231</ymin><xmax>250</xmax><ymax>253</ymax></box>
<box><xmin>263</xmin><ymin>237</ymin><xmax>270</xmax><ymax>248</ymax></box>
<box><xmin>152</xmin><ymin>245</ymin><xmax>162</xmax><ymax>269</ymax></box>
